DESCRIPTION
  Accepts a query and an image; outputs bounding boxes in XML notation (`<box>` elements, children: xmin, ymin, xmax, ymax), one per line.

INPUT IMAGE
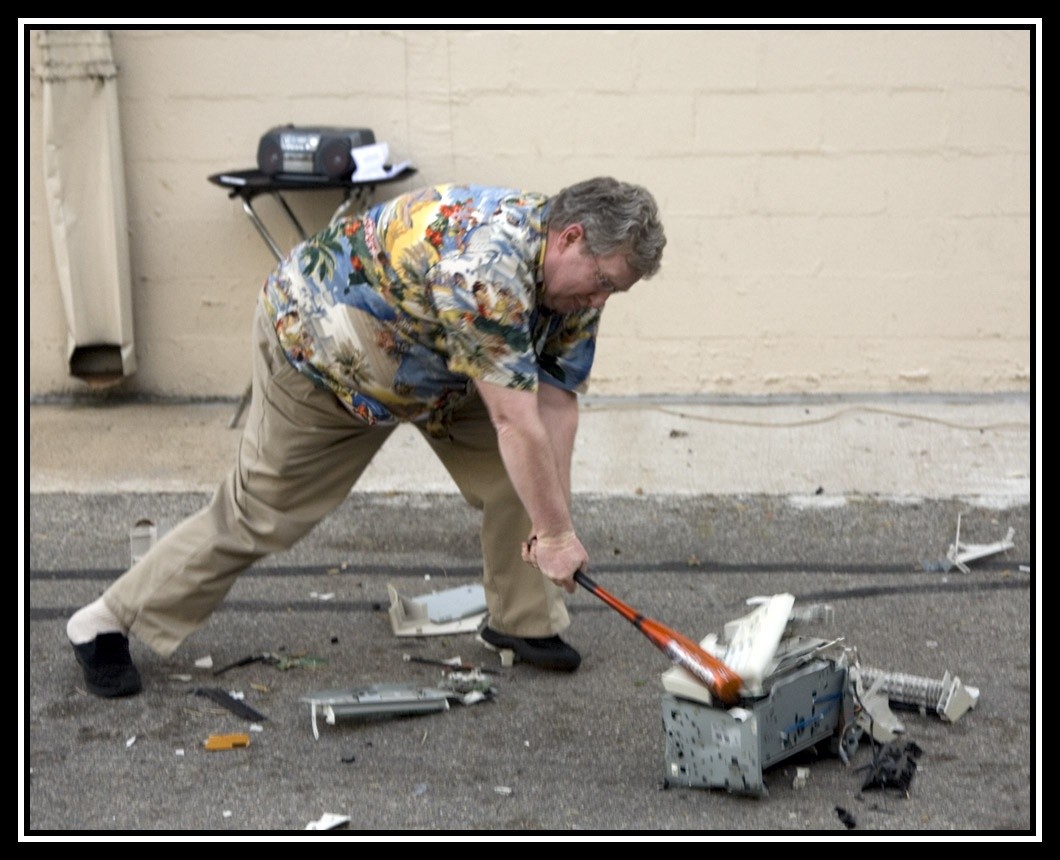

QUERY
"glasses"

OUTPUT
<box><xmin>584</xmin><ymin>236</ymin><xmax>615</xmax><ymax>292</ymax></box>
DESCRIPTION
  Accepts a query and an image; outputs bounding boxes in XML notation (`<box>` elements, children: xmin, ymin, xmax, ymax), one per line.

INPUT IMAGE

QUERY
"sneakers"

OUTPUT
<box><xmin>480</xmin><ymin>627</ymin><xmax>580</xmax><ymax>670</ymax></box>
<box><xmin>70</xmin><ymin>631</ymin><xmax>141</xmax><ymax>696</ymax></box>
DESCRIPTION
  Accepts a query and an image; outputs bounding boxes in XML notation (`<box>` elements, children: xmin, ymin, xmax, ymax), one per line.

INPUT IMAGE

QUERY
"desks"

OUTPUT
<box><xmin>207</xmin><ymin>166</ymin><xmax>418</xmax><ymax>427</ymax></box>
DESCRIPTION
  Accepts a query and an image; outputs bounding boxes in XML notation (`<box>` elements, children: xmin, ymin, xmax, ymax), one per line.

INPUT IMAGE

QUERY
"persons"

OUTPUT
<box><xmin>65</xmin><ymin>176</ymin><xmax>667</xmax><ymax>699</ymax></box>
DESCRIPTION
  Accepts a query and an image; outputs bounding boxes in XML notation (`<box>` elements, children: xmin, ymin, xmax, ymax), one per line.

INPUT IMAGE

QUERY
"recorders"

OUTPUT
<box><xmin>257</xmin><ymin>126</ymin><xmax>375</xmax><ymax>177</ymax></box>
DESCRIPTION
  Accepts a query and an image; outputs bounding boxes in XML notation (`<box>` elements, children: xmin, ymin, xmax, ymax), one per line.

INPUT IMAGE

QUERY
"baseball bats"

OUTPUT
<box><xmin>524</xmin><ymin>533</ymin><xmax>743</xmax><ymax>701</ymax></box>
